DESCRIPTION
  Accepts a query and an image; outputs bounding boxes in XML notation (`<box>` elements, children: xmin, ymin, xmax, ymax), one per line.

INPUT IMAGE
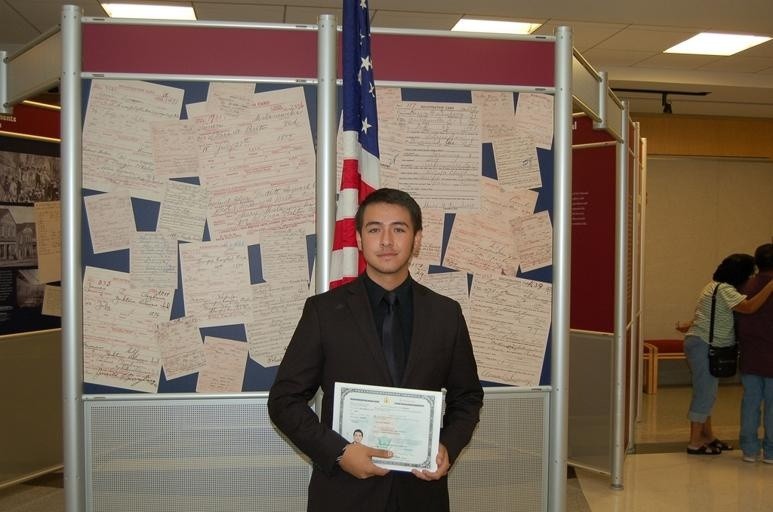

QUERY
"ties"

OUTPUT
<box><xmin>383</xmin><ymin>292</ymin><xmax>406</xmax><ymax>389</ymax></box>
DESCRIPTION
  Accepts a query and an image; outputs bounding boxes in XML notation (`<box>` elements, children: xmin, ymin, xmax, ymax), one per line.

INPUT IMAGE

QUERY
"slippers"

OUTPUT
<box><xmin>687</xmin><ymin>439</ymin><xmax>734</xmax><ymax>455</ymax></box>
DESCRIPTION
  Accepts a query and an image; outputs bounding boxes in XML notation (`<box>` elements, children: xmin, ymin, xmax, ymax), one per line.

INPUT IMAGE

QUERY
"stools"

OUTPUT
<box><xmin>643</xmin><ymin>339</ymin><xmax>692</xmax><ymax>394</ymax></box>
<box><xmin>643</xmin><ymin>343</ymin><xmax>654</xmax><ymax>394</ymax></box>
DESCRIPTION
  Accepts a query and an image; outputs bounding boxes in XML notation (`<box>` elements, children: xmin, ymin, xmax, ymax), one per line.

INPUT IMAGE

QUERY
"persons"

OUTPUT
<box><xmin>681</xmin><ymin>253</ymin><xmax>772</xmax><ymax>455</ymax></box>
<box><xmin>1</xmin><ymin>165</ymin><xmax>59</xmax><ymax>205</ymax></box>
<box><xmin>351</xmin><ymin>430</ymin><xmax>363</xmax><ymax>446</ymax></box>
<box><xmin>731</xmin><ymin>243</ymin><xmax>772</xmax><ymax>465</ymax></box>
<box><xmin>266</xmin><ymin>185</ymin><xmax>485</xmax><ymax>512</ymax></box>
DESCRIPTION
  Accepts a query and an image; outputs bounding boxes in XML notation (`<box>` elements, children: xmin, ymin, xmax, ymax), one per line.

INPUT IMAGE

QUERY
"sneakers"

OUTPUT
<box><xmin>744</xmin><ymin>454</ymin><xmax>773</xmax><ymax>464</ymax></box>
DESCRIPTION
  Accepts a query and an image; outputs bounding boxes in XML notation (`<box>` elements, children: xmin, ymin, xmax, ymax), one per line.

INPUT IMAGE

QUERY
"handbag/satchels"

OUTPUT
<box><xmin>708</xmin><ymin>344</ymin><xmax>737</xmax><ymax>378</ymax></box>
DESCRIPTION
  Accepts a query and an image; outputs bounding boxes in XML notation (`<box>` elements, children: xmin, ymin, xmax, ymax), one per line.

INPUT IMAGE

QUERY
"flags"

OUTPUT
<box><xmin>329</xmin><ymin>0</ymin><xmax>380</xmax><ymax>290</ymax></box>
